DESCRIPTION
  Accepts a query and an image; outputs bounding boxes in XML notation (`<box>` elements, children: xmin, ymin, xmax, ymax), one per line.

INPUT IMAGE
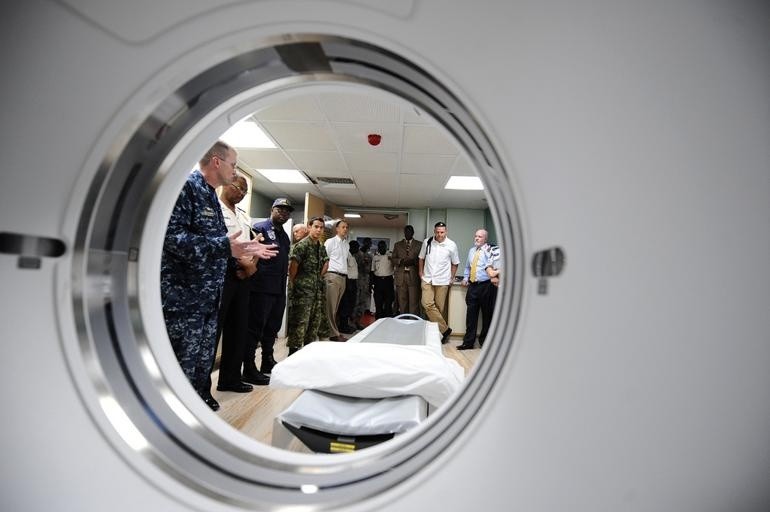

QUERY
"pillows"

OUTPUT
<box><xmin>264</xmin><ymin>334</ymin><xmax>465</xmax><ymax>408</ymax></box>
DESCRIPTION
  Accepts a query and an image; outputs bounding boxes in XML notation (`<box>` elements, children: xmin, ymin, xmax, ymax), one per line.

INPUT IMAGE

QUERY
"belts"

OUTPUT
<box><xmin>329</xmin><ymin>271</ymin><xmax>347</xmax><ymax>277</ymax></box>
<box><xmin>471</xmin><ymin>282</ymin><xmax>492</xmax><ymax>286</ymax></box>
<box><xmin>375</xmin><ymin>276</ymin><xmax>392</xmax><ymax>281</ymax></box>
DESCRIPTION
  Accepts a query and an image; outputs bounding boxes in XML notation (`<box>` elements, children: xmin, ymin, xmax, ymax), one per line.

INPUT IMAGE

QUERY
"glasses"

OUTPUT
<box><xmin>214</xmin><ymin>154</ymin><xmax>237</xmax><ymax>170</ymax></box>
<box><xmin>232</xmin><ymin>184</ymin><xmax>248</xmax><ymax>196</ymax></box>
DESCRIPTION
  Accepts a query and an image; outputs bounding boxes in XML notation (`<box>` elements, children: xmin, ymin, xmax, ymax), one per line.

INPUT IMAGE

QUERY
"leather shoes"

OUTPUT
<box><xmin>330</xmin><ymin>321</ymin><xmax>362</xmax><ymax>341</ymax></box>
<box><xmin>217</xmin><ymin>382</ymin><xmax>254</xmax><ymax>391</ymax></box>
<box><xmin>456</xmin><ymin>342</ymin><xmax>473</xmax><ymax>349</ymax></box>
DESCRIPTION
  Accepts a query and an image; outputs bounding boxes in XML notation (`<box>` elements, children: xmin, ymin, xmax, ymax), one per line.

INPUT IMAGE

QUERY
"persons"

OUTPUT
<box><xmin>286</xmin><ymin>215</ymin><xmax>500</xmax><ymax>358</ymax></box>
<box><xmin>240</xmin><ymin>198</ymin><xmax>294</xmax><ymax>386</ymax></box>
<box><xmin>200</xmin><ymin>174</ymin><xmax>260</xmax><ymax>410</ymax></box>
<box><xmin>160</xmin><ymin>141</ymin><xmax>280</xmax><ymax>411</ymax></box>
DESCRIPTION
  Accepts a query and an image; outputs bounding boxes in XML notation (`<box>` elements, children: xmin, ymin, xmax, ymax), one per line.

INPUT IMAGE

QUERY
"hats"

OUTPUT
<box><xmin>435</xmin><ymin>222</ymin><xmax>445</xmax><ymax>227</ymax></box>
<box><xmin>272</xmin><ymin>197</ymin><xmax>295</xmax><ymax>212</ymax></box>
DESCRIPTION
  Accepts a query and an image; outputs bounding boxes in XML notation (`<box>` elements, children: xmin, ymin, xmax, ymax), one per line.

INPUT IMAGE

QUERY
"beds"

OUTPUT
<box><xmin>283</xmin><ymin>314</ymin><xmax>451</xmax><ymax>454</ymax></box>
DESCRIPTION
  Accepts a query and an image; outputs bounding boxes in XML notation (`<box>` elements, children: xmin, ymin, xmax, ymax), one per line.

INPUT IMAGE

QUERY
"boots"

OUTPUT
<box><xmin>242</xmin><ymin>359</ymin><xmax>271</xmax><ymax>386</ymax></box>
<box><xmin>203</xmin><ymin>378</ymin><xmax>219</xmax><ymax>409</ymax></box>
<box><xmin>261</xmin><ymin>352</ymin><xmax>279</xmax><ymax>373</ymax></box>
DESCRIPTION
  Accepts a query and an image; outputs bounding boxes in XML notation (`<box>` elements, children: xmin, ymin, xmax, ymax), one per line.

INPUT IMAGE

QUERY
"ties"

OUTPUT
<box><xmin>469</xmin><ymin>248</ymin><xmax>479</xmax><ymax>283</ymax></box>
<box><xmin>406</xmin><ymin>240</ymin><xmax>411</xmax><ymax>257</ymax></box>
